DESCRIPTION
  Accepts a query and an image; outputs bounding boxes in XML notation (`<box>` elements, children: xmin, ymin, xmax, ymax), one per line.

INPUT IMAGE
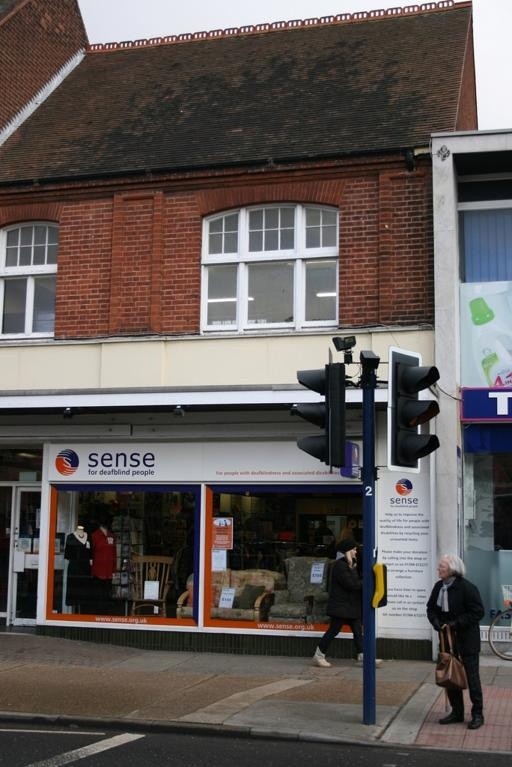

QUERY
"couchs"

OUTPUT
<box><xmin>176</xmin><ymin>568</ymin><xmax>287</xmax><ymax>622</ymax></box>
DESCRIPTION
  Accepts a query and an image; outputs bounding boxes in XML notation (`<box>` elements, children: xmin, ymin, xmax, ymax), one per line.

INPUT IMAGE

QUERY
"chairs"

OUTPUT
<box><xmin>130</xmin><ymin>555</ymin><xmax>173</xmax><ymax>617</ymax></box>
<box><xmin>268</xmin><ymin>556</ymin><xmax>335</xmax><ymax>624</ymax></box>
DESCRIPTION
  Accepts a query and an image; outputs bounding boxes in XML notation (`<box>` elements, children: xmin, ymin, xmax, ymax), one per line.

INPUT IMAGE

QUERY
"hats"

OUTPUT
<box><xmin>336</xmin><ymin>538</ymin><xmax>357</xmax><ymax>553</ymax></box>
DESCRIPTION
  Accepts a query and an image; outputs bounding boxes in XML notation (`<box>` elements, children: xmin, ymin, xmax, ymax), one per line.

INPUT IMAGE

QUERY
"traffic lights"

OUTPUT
<box><xmin>385</xmin><ymin>350</ymin><xmax>440</xmax><ymax>472</ymax></box>
<box><xmin>292</xmin><ymin>359</ymin><xmax>348</xmax><ymax>471</ymax></box>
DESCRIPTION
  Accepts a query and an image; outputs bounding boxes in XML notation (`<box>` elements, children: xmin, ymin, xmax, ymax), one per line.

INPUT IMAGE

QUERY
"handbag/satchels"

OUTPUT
<box><xmin>434</xmin><ymin>651</ymin><xmax>468</xmax><ymax>689</ymax></box>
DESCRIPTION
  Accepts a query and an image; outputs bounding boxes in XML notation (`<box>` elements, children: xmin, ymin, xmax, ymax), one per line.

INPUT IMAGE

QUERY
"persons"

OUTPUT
<box><xmin>311</xmin><ymin>539</ymin><xmax>384</xmax><ymax>667</ymax></box>
<box><xmin>91</xmin><ymin>519</ymin><xmax>119</xmax><ymax>612</ymax></box>
<box><xmin>425</xmin><ymin>552</ymin><xmax>484</xmax><ymax>729</ymax></box>
<box><xmin>314</xmin><ymin>518</ymin><xmax>336</xmax><ymax>549</ymax></box>
<box><xmin>61</xmin><ymin>524</ymin><xmax>94</xmax><ymax>613</ymax></box>
<box><xmin>340</xmin><ymin>516</ymin><xmax>362</xmax><ymax>541</ymax></box>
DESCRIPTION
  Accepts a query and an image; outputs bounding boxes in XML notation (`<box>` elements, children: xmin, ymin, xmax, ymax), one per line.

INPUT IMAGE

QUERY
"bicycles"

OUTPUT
<box><xmin>487</xmin><ymin>584</ymin><xmax>512</xmax><ymax>661</ymax></box>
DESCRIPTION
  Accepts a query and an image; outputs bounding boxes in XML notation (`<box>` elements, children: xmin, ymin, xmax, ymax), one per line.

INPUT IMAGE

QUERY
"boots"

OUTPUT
<box><xmin>358</xmin><ymin>652</ymin><xmax>383</xmax><ymax>668</ymax></box>
<box><xmin>312</xmin><ymin>646</ymin><xmax>331</xmax><ymax>668</ymax></box>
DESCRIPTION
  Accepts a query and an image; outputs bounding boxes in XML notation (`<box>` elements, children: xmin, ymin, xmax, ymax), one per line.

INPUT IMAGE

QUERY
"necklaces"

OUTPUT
<box><xmin>78</xmin><ymin>532</ymin><xmax>85</xmax><ymax>538</ymax></box>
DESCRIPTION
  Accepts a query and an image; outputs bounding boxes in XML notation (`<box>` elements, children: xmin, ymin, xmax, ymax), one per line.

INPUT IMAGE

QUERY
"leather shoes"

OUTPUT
<box><xmin>439</xmin><ymin>708</ymin><xmax>465</xmax><ymax>724</ymax></box>
<box><xmin>467</xmin><ymin>710</ymin><xmax>484</xmax><ymax>729</ymax></box>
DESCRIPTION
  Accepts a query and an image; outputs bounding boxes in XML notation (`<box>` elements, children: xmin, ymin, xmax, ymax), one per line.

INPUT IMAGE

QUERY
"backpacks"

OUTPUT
<box><xmin>326</xmin><ymin>561</ymin><xmax>338</xmax><ymax>593</ymax></box>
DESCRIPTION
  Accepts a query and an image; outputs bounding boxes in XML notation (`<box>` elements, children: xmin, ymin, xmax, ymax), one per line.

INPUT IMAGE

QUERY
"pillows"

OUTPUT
<box><xmin>187</xmin><ymin>584</ymin><xmax>221</xmax><ymax>607</ymax></box>
<box><xmin>238</xmin><ymin>583</ymin><xmax>265</xmax><ymax>609</ymax></box>
